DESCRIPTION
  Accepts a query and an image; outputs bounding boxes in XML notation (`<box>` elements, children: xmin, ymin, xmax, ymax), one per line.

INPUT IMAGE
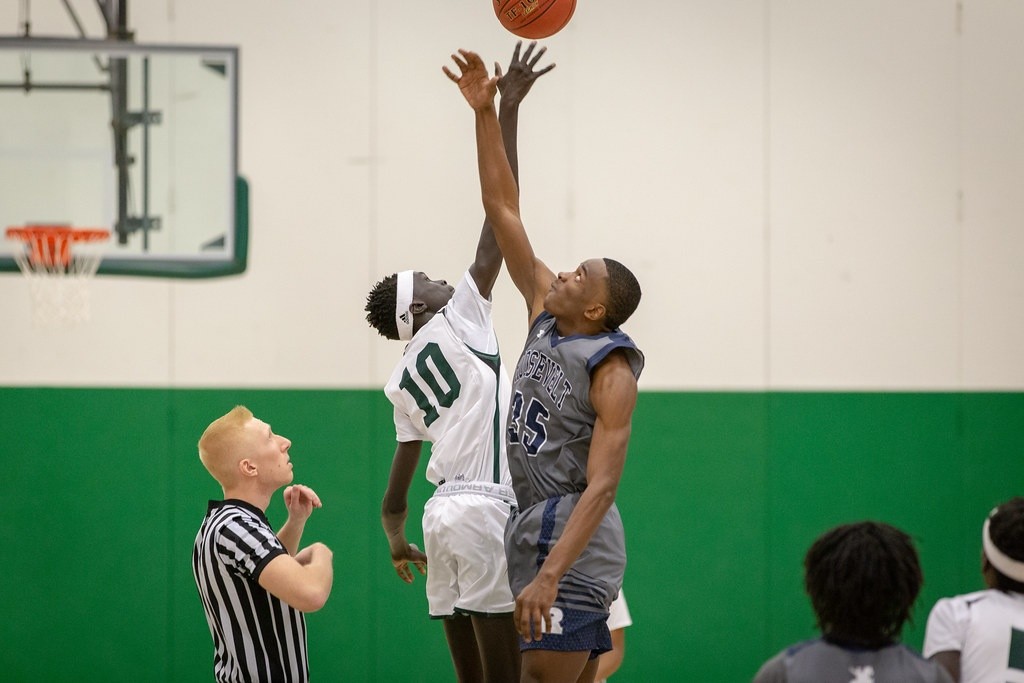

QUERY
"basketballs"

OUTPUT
<box><xmin>491</xmin><ymin>0</ymin><xmax>578</xmax><ymax>40</ymax></box>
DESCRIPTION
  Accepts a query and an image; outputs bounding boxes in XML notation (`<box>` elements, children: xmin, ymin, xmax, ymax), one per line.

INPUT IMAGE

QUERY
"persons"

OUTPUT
<box><xmin>595</xmin><ymin>585</ymin><xmax>634</xmax><ymax>683</ymax></box>
<box><xmin>364</xmin><ymin>40</ymin><xmax>556</xmax><ymax>683</ymax></box>
<box><xmin>750</xmin><ymin>521</ymin><xmax>957</xmax><ymax>683</ymax></box>
<box><xmin>441</xmin><ymin>48</ymin><xmax>648</xmax><ymax>683</ymax></box>
<box><xmin>921</xmin><ymin>497</ymin><xmax>1024</xmax><ymax>683</ymax></box>
<box><xmin>191</xmin><ymin>404</ymin><xmax>335</xmax><ymax>683</ymax></box>
